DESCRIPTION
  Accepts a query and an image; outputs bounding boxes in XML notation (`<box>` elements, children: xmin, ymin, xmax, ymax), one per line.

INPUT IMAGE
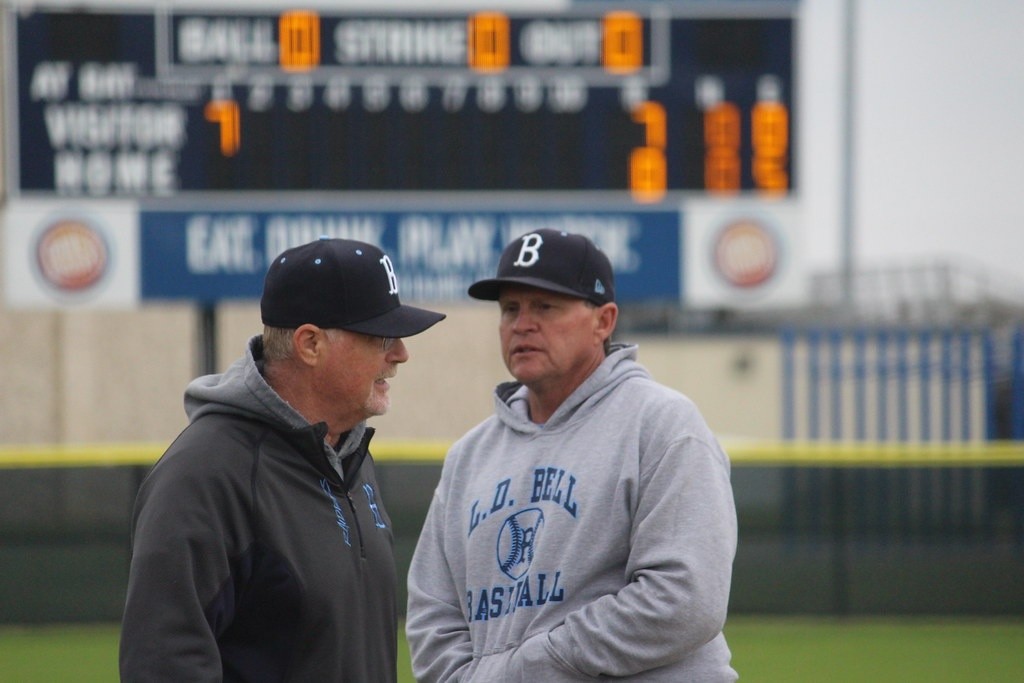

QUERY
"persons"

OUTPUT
<box><xmin>118</xmin><ymin>238</ymin><xmax>445</xmax><ymax>682</ymax></box>
<box><xmin>404</xmin><ymin>227</ymin><xmax>742</xmax><ymax>683</ymax></box>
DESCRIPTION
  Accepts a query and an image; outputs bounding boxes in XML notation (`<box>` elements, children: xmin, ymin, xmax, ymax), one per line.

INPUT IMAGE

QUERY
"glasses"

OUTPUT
<box><xmin>381</xmin><ymin>336</ymin><xmax>398</xmax><ymax>351</ymax></box>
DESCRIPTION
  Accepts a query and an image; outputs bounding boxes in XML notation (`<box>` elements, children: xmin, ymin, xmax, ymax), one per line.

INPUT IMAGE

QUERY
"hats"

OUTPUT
<box><xmin>261</xmin><ymin>236</ymin><xmax>446</xmax><ymax>339</ymax></box>
<box><xmin>468</xmin><ymin>229</ymin><xmax>616</xmax><ymax>307</ymax></box>
